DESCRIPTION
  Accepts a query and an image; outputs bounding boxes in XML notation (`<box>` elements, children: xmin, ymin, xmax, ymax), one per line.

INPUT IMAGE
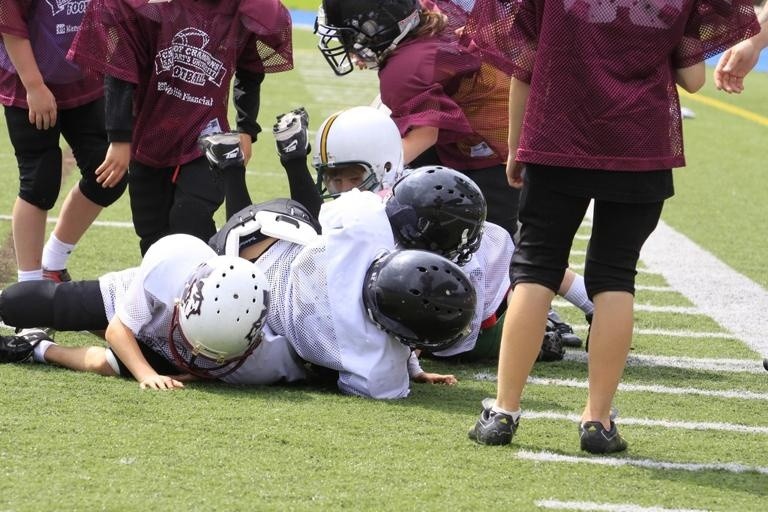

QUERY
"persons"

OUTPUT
<box><xmin>0</xmin><ymin>1</ymin><xmax>129</xmax><ymax>286</ymax></box>
<box><xmin>197</xmin><ymin>107</ymin><xmax>477</xmax><ymax>400</ymax></box>
<box><xmin>0</xmin><ymin>234</ymin><xmax>306</xmax><ymax>391</ymax></box>
<box><xmin>313</xmin><ymin>0</ymin><xmax>596</xmax><ymax>351</ymax></box>
<box><xmin>65</xmin><ymin>1</ymin><xmax>293</xmax><ymax>262</ymax></box>
<box><xmin>714</xmin><ymin>1</ymin><xmax>767</xmax><ymax>94</ymax></box>
<box><xmin>382</xmin><ymin>166</ymin><xmax>565</xmax><ymax>364</ymax></box>
<box><xmin>456</xmin><ymin>1</ymin><xmax>761</xmax><ymax>455</ymax></box>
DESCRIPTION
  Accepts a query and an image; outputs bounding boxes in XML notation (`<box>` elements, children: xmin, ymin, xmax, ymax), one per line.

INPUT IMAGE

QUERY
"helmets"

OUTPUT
<box><xmin>313</xmin><ymin>0</ymin><xmax>422</xmax><ymax>76</ymax></box>
<box><xmin>362</xmin><ymin>248</ymin><xmax>478</xmax><ymax>352</ymax></box>
<box><xmin>384</xmin><ymin>164</ymin><xmax>488</xmax><ymax>269</ymax></box>
<box><xmin>168</xmin><ymin>254</ymin><xmax>272</xmax><ymax>380</ymax></box>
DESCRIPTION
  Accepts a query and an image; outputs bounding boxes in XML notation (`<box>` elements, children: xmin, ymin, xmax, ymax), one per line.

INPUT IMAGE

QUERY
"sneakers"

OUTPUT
<box><xmin>467</xmin><ymin>397</ymin><xmax>521</xmax><ymax>446</ymax></box>
<box><xmin>578</xmin><ymin>420</ymin><xmax>628</xmax><ymax>455</ymax></box>
<box><xmin>0</xmin><ymin>327</ymin><xmax>54</xmax><ymax>366</ymax></box>
<box><xmin>272</xmin><ymin>106</ymin><xmax>311</xmax><ymax>156</ymax></box>
<box><xmin>537</xmin><ymin>326</ymin><xmax>565</xmax><ymax>362</ymax></box>
<box><xmin>544</xmin><ymin>304</ymin><xmax>584</xmax><ymax>349</ymax></box>
<box><xmin>194</xmin><ymin>129</ymin><xmax>246</xmax><ymax>172</ymax></box>
<box><xmin>42</xmin><ymin>268</ymin><xmax>73</xmax><ymax>283</ymax></box>
